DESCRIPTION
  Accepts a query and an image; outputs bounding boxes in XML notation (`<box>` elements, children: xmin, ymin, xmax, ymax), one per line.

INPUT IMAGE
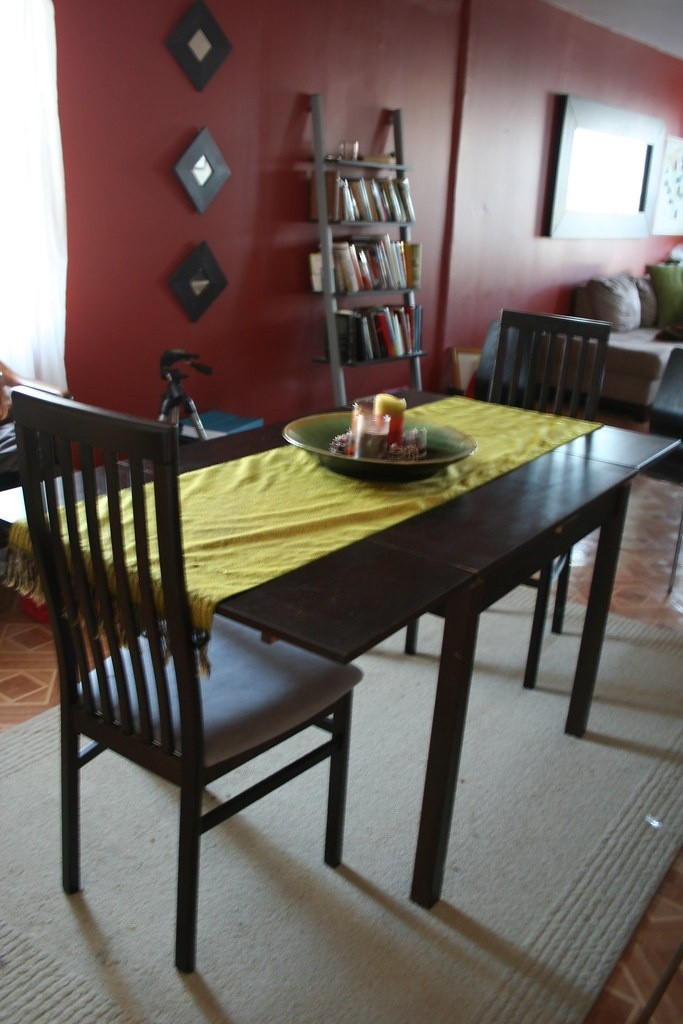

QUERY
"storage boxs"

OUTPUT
<box><xmin>175</xmin><ymin>408</ymin><xmax>264</xmax><ymax>441</ymax></box>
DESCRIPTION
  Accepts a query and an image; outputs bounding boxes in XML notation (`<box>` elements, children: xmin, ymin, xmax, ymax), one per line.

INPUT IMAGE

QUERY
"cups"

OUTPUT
<box><xmin>339</xmin><ymin>141</ymin><xmax>359</xmax><ymax>160</ymax></box>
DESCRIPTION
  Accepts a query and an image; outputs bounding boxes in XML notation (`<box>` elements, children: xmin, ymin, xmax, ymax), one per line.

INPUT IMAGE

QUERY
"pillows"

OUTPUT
<box><xmin>647</xmin><ymin>263</ymin><xmax>683</xmax><ymax>333</ymax></box>
<box><xmin>633</xmin><ymin>277</ymin><xmax>658</xmax><ymax>328</ymax></box>
<box><xmin>586</xmin><ymin>273</ymin><xmax>641</xmax><ymax>331</ymax></box>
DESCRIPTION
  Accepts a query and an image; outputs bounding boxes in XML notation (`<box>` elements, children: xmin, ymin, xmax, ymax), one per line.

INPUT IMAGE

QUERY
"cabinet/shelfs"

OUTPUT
<box><xmin>309</xmin><ymin>94</ymin><xmax>426</xmax><ymax>408</ymax></box>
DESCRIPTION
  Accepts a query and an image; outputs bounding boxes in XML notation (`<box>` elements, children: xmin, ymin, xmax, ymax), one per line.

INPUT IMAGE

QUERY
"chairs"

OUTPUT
<box><xmin>404</xmin><ymin>309</ymin><xmax>613</xmax><ymax>689</ymax></box>
<box><xmin>9</xmin><ymin>384</ymin><xmax>361</xmax><ymax>972</ymax></box>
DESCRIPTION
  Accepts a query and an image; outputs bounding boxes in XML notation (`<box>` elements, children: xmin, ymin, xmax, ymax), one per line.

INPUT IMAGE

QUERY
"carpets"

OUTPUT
<box><xmin>0</xmin><ymin>585</ymin><xmax>683</xmax><ymax>1024</ymax></box>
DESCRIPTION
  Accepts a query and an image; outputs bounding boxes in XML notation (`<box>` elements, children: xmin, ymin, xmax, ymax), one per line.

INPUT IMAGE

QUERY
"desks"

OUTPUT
<box><xmin>0</xmin><ymin>386</ymin><xmax>683</xmax><ymax>910</ymax></box>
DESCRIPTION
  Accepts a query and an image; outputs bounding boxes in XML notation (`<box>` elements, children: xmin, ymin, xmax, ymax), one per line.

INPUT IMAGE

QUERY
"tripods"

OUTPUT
<box><xmin>129</xmin><ymin>385</ymin><xmax>224</xmax><ymax>488</ymax></box>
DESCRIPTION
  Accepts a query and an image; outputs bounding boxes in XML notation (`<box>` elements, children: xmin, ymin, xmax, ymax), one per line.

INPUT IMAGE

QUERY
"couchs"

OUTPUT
<box><xmin>472</xmin><ymin>258</ymin><xmax>683</xmax><ymax>481</ymax></box>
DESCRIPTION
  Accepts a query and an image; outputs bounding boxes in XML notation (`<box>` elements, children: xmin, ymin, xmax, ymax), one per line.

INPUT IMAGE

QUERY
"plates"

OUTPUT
<box><xmin>280</xmin><ymin>411</ymin><xmax>479</xmax><ymax>482</ymax></box>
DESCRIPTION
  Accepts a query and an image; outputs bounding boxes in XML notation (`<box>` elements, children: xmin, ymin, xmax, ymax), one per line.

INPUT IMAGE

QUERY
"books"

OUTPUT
<box><xmin>310</xmin><ymin>170</ymin><xmax>415</xmax><ymax>223</ymax></box>
<box><xmin>325</xmin><ymin>305</ymin><xmax>422</xmax><ymax>367</ymax></box>
<box><xmin>309</xmin><ymin>233</ymin><xmax>423</xmax><ymax>292</ymax></box>
